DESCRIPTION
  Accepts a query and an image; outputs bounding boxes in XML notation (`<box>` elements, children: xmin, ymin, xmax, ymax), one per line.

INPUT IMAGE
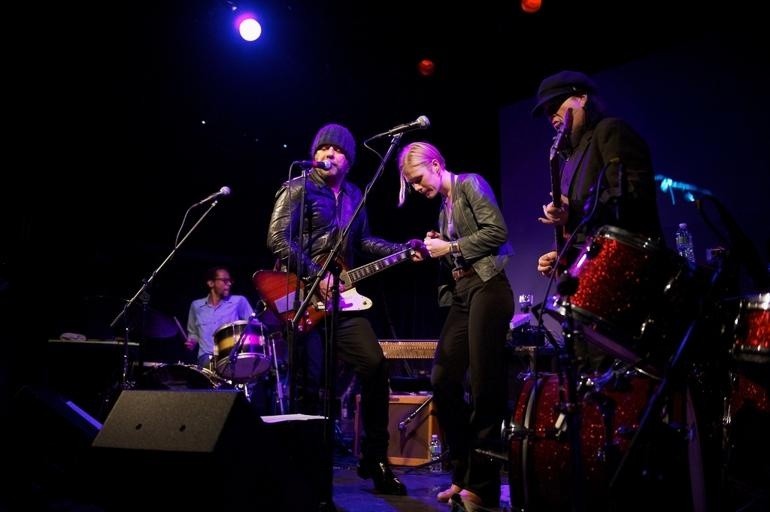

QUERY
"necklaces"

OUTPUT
<box><xmin>440</xmin><ymin>173</ymin><xmax>456</xmax><ymax>225</ymax></box>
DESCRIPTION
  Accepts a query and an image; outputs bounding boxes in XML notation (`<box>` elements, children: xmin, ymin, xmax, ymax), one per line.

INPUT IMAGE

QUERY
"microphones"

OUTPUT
<box><xmin>200</xmin><ymin>186</ymin><xmax>232</xmax><ymax>203</ymax></box>
<box><xmin>660</xmin><ymin>177</ymin><xmax>713</xmax><ymax>196</ymax></box>
<box><xmin>297</xmin><ymin>160</ymin><xmax>332</xmax><ymax>171</ymax></box>
<box><xmin>614</xmin><ymin>164</ymin><xmax>629</xmax><ymax>220</ymax></box>
<box><xmin>388</xmin><ymin>115</ymin><xmax>430</xmax><ymax>136</ymax></box>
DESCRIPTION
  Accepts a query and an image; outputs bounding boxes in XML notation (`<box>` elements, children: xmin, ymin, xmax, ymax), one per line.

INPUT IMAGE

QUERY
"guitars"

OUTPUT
<box><xmin>252</xmin><ymin>246</ymin><xmax>417</xmax><ymax>338</ymax></box>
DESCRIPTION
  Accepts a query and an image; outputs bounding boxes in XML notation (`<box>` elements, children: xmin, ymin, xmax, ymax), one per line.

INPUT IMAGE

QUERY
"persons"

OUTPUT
<box><xmin>397</xmin><ymin>141</ymin><xmax>514</xmax><ymax>510</ymax></box>
<box><xmin>184</xmin><ymin>266</ymin><xmax>258</xmax><ymax>368</ymax></box>
<box><xmin>528</xmin><ymin>71</ymin><xmax>662</xmax><ymax>376</ymax></box>
<box><xmin>266</xmin><ymin>122</ymin><xmax>425</xmax><ymax>494</ymax></box>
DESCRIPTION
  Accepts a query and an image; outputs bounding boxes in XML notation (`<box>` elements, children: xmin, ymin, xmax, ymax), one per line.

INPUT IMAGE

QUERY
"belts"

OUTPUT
<box><xmin>451</xmin><ymin>267</ymin><xmax>477</xmax><ymax>282</ymax></box>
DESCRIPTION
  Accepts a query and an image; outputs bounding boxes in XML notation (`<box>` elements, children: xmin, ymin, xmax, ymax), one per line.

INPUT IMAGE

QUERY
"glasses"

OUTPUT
<box><xmin>214</xmin><ymin>278</ymin><xmax>235</xmax><ymax>285</ymax></box>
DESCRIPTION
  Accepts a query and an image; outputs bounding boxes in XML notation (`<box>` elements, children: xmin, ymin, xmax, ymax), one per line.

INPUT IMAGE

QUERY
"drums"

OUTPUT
<box><xmin>268</xmin><ymin>331</ymin><xmax>291</xmax><ymax>370</ymax></box>
<box><xmin>554</xmin><ymin>224</ymin><xmax>702</xmax><ymax>369</ymax></box>
<box><xmin>139</xmin><ymin>362</ymin><xmax>234</xmax><ymax>391</ymax></box>
<box><xmin>213</xmin><ymin>318</ymin><xmax>273</xmax><ymax>382</ymax></box>
<box><xmin>725</xmin><ymin>299</ymin><xmax>768</xmax><ymax>365</ymax></box>
<box><xmin>717</xmin><ymin>354</ymin><xmax>770</xmax><ymax>473</ymax></box>
<box><xmin>507</xmin><ymin>365</ymin><xmax>708</xmax><ymax>510</ymax></box>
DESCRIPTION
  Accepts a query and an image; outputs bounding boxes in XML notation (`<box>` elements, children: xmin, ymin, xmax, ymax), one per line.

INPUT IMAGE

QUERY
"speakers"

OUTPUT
<box><xmin>355</xmin><ymin>395</ymin><xmax>442</xmax><ymax>467</ymax></box>
<box><xmin>92</xmin><ymin>391</ymin><xmax>298</xmax><ymax>512</ymax></box>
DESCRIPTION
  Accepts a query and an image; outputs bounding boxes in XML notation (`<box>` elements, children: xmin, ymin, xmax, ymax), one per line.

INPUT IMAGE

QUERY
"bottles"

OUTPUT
<box><xmin>428</xmin><ymin>433</ymin><xmax>444</xmax><ymax>472</ymax></box>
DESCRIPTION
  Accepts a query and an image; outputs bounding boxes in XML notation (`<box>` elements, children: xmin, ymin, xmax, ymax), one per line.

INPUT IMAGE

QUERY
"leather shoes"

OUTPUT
<box><xmin>356</xmin><ymin>452</ymin><xmax>407</xmax><ymax>496</ymax></box>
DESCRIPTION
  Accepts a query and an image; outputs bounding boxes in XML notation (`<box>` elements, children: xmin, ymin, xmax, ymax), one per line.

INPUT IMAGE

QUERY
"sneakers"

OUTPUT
<box><xmin>448</xmin><ymin>493</ymin><xmax>482</xmax><ymax>506</ymax></box>
<box><xmin>434</xmin><ymin>483</ymin><xmax>463</xmax><ymax>503</ymax></box>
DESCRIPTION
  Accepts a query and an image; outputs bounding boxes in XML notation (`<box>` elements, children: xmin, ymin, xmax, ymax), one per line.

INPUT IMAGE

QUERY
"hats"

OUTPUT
<box><xmin>530</xmin><ymin>69</ymin><xmax>602</xmax><ymax>118</ymax></box>
<box><xmin>310</xmin><ymin>123</ymin><xmax>358</xmax><ymax>173</ymax></box>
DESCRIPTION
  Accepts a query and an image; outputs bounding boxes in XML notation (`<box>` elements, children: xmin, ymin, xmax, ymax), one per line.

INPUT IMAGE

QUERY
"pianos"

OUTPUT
<box><xmin>378</xmin><ymin>341</ymin><xmax>439</xmax><ymax>359</ymax></box>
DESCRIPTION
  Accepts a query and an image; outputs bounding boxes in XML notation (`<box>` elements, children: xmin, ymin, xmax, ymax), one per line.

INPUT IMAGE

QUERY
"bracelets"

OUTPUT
<box><xmin>450</xmin><ymin>240</ymin><xmax>458</xmax><ymax>255</ymax></box>
